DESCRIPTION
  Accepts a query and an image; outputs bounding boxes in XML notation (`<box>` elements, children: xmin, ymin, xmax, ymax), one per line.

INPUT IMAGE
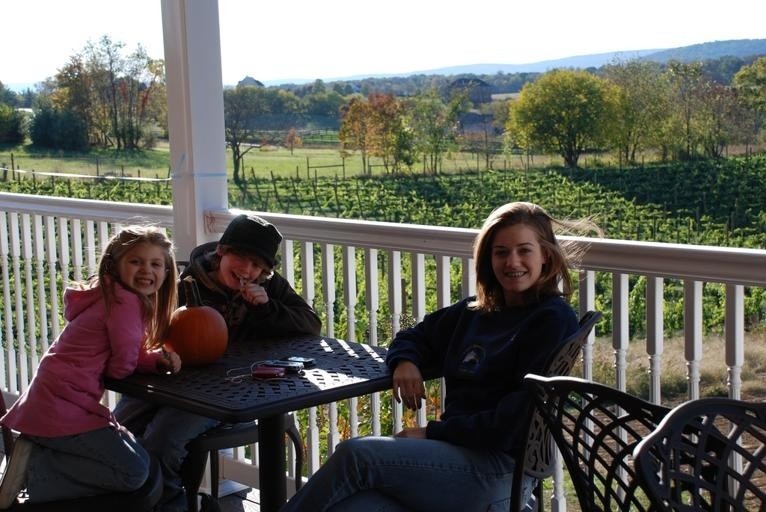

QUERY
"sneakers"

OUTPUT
<box><xmin>0</xmin><ymin>433</ymin><xmax>33</xmax><ymax>510</ymax></box>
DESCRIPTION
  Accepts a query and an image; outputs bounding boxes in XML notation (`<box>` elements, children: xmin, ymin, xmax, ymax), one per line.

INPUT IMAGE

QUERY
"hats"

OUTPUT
<box><xmin>219</xmin><ymin>214</ymin><xmax>284</xmax><ymax>268</ymax></box>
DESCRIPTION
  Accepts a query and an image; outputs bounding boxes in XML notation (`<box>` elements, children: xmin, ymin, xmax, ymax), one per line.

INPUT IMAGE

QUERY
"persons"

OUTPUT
<box><xmin>279</xmin><ymin>201</ymin><xmax>579</xmax><ymax>512</ymax></box>
<box><xmin>0</xmin><ymin>221</ymin><xmax>182</xmax><ymax>510</ymax></box>
<box><xmin>109</xmin><ymin>214</ymin><xmax>323</xmax><ymax>511</ymax></box>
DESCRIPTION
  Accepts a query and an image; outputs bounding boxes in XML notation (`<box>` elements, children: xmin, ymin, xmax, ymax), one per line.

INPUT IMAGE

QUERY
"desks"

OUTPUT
<box><xmin>96</xmin><ymin>322</ymin><xmax>444</xmax><ymax>509</ymax></box>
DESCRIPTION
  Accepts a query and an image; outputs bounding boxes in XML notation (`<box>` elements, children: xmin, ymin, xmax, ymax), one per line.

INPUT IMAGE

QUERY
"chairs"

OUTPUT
<box><xmin>1</xmin><ymin>386</ymin><xmax>188</xmax><ymax>512</ymax></box>
<box><xmin>171</xmin><ymin>258</ymin><xmax>306</xmax><ymax>509</ymax></box>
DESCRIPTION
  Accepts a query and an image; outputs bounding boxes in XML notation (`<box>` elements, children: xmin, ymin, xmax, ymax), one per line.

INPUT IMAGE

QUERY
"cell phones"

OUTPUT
<box><xmin>252</xmin><ymin>367</ymin><xmax>286</xmax><ymax>378</ymax></box>
<box><xmin>265</xmin><ymin>359</ymin><xmax>304</xmax><ymax>372</ymax></box>
<box><xmin>280</xmin><ymin>355</ymin><xmax>316</xmax><ymax>367</ymax></box>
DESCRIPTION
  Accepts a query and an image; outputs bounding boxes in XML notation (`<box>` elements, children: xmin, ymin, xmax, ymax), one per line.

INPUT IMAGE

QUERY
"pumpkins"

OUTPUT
<box><xmin>168</xmin><ymin>276</ymin><xmax>229</xmax><ymax>366</ymax></box>
